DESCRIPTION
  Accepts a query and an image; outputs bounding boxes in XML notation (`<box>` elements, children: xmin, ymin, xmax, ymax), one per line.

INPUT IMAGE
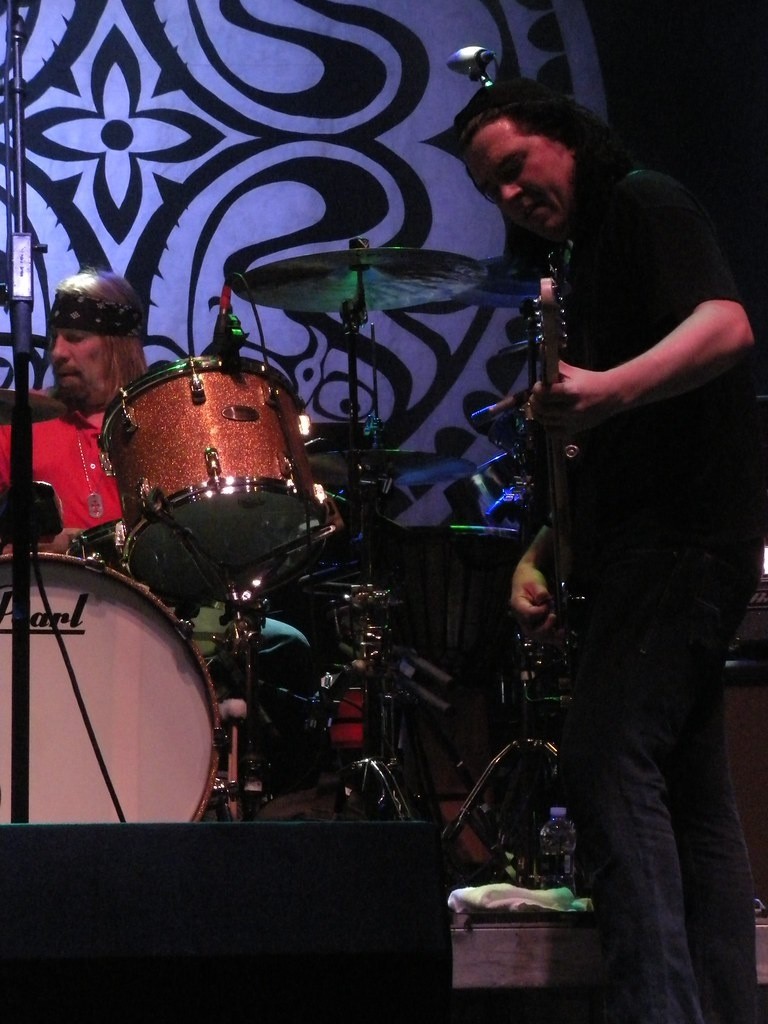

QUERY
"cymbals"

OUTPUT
<box><xmin>0</xmin><ymin>386</ymin><xmax>67</xmax><ymax>426</ymax></box>
<box><xmin>230</xmin><ymin>232</ymin><xmax>490</xmax><ymax>315</ymax></box>
<box><xmin>306</xmin><ymin>444</ymin><xmax>479</xmax><ymax>490</ymax></box>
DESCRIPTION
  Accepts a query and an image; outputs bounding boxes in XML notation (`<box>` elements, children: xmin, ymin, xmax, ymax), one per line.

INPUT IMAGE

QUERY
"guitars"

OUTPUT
<box><xmin>522</xmin><ymin>273</ymin><xmax>583</xmax><ymax>651</ymax></box>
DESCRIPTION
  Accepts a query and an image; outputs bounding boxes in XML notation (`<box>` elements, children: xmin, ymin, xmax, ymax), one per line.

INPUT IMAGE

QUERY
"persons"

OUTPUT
<box><xmin>0</xmin><ymin>270</ymin><xmax>309</xmax><ymax>795</ymax></box>
<box><xmin>457</xmin><ymin>72</ymin><xmax>766</xmax><ymax>1023</ymax></box>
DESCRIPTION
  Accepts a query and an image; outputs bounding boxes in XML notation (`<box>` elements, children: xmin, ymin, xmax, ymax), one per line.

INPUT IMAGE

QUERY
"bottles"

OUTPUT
<box><xmin>752</xmin><ymin>899</ymin><xmax>768</xmax><ymax>989</ymax></box>
<box><xmin>538</xmin><ymin>807</ymin><xmax>576</xmax><ymax>890</ymax></box>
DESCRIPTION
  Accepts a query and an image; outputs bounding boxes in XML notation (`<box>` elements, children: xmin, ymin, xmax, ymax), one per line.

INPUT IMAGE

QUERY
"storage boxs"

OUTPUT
<box><xmin>451</xmin><ymin>912</ymin><xmax>768</xmax><ymax>993</ymax></box>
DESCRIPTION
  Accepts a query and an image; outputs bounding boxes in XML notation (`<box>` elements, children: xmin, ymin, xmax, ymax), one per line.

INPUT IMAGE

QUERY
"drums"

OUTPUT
<box><xmin>0</xmin><ymin>549</ymin><xmax>225</xmax><ymax>826</ymax></box>
<box><xmin>97</xmin><ymin>353</ymin><xmax>326</xmax><ymax>605</ymax></box>
